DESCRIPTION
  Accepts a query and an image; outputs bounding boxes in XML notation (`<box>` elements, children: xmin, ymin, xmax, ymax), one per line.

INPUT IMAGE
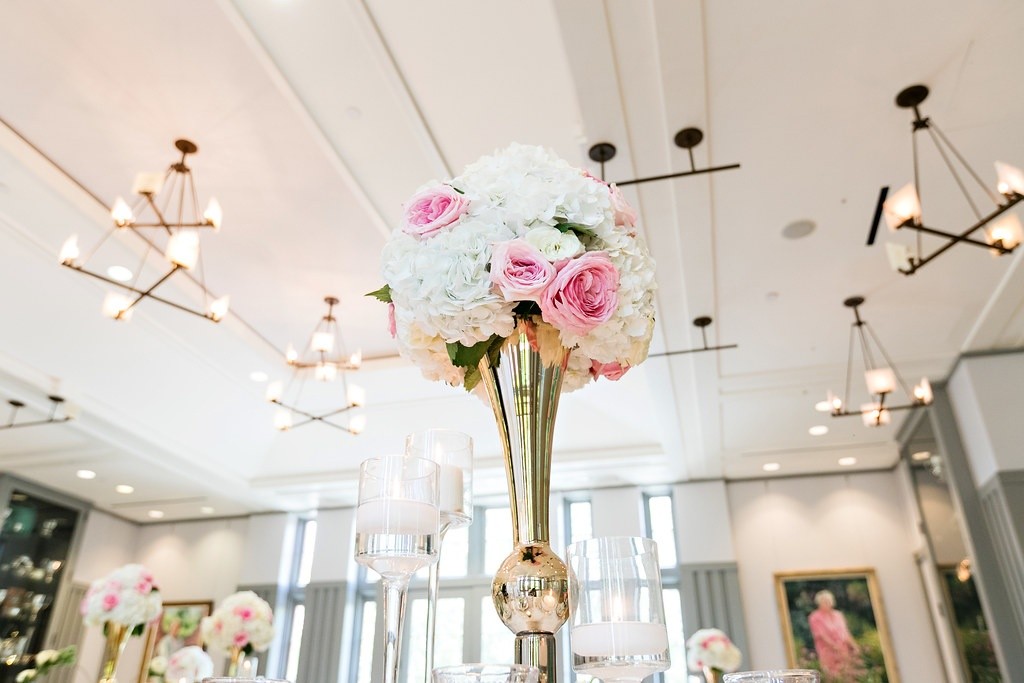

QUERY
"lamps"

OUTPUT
<box><xmin>823</xmin><ymin>297</ymin><xmax>934</xmax><ymax>429</ymax></box>
<box><xmin>881</xmin><ymin>83</ymin><xmax>1024</xmax><ymax>276</ymax></box>
<box><xmin>56</xmin><ymin>138</ymin><xmax>231</xmax><ymax>324</ymax></box>
<box><xmin>269</xmin><ymin>297</ymin><xmax>362</xmax><ymax>436</ymax></box>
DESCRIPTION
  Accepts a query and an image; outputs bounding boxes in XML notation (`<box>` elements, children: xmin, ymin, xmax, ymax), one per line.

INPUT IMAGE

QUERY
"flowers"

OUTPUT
<box><xmin>362</xmin><ymin>140</ymin><xmax>655</xmax><ymax>394</ymax></box>
<box><xmin>686</xmin><ymin>628</ymin><xmax>742</xmax><ymax>674</ymax></box>
<box><xmin>16</xmin><ymin>644</ymin><xmax>78</xmax><ymax>683</ymax></box>
<box><xmin>79</xmin><ymin>564</ymin><xmax>163</xmax><ymax>627</ymax></box>
<box><xmin>200</xmin><ymin>590</ymin><xmax>276</xmax><ymax>657</ymax></box>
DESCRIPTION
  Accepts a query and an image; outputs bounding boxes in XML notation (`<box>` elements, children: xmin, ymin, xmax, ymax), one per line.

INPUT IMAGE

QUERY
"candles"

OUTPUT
<box><xmin>354</xmin><ymin>455</ymin><xmax>441</xmax><ymax>573</ymax></box>
<box><xmin>566</xmin><ymin>536</ymin><xmax>671</xmax><ymax>679</ymax></box>
<box><xmin>399</xmin><ymin>430</ymin><xmax>474</xmax><ymax>530</ymax></box>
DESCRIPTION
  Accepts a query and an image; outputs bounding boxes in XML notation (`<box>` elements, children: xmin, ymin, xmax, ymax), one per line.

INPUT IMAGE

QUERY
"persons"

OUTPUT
<box><xmin>809</xmin><ymin>590</ymin><xmax>860</xmax><ymax>675</ymax></box>
<box><xmin>164</xmin><ymin>627</ymin><xmax>213</xmax><ymax>683</ymax></box>
<box><xmin>158</xmin><ymin>619</ymin><xmax>184</xmax><ymax>657</ymax></box>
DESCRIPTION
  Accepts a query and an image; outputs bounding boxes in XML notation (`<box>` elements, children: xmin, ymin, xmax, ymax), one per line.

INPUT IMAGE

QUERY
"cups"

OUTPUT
<box><xmin>722</xmin><ymin>668</ymin><xmax>820</xmax><ymax>683</ymax></box>
<box><xmin>430</xmin><ymin>663</ymin><xmax>540</xmax><ymax>683</ymax></box>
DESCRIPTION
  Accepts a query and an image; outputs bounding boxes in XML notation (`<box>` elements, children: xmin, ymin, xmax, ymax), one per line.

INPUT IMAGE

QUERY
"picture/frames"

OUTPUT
<box><xmin>773</xmin><ymin>567</ymin><xmax>901</xmax><ymax>683</ymax></box>
<box><xmin>137</xmin><ymin>600</ymin><xmax>214</xmax><ymax>683</ymax></box>
